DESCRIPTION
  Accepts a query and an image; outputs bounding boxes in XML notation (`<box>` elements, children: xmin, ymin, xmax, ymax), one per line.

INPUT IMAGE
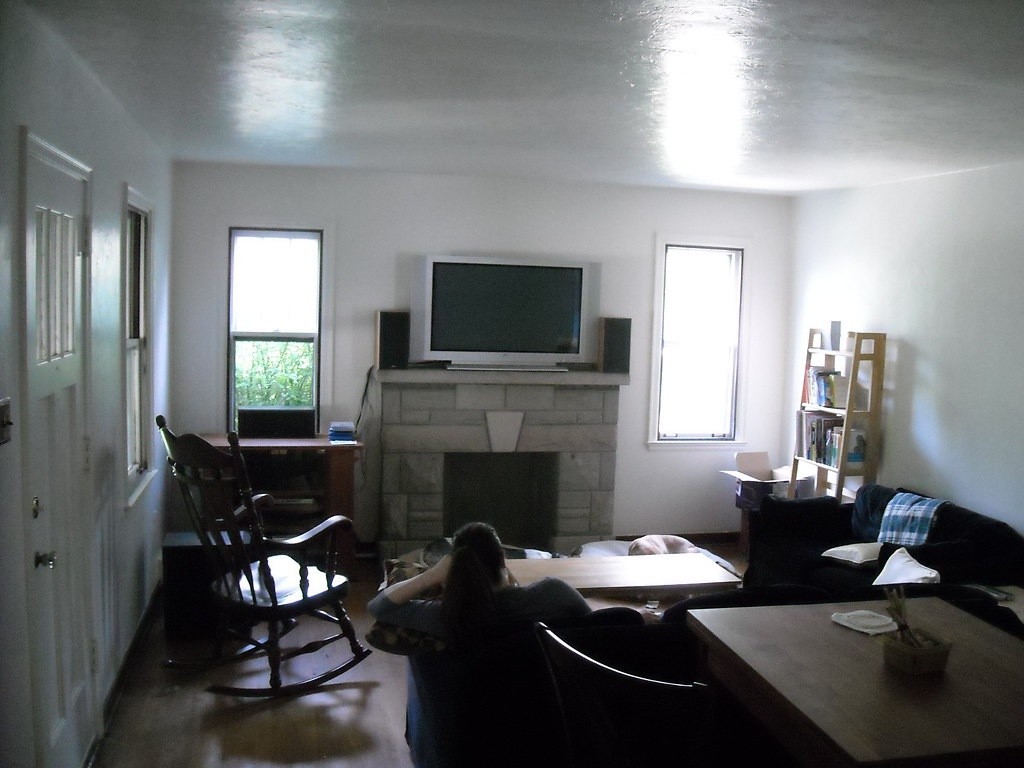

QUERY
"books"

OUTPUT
<box><xmin>800</xmin><ymin>365</ymin><xmax>867</xmax><ymax>469</ymax></box>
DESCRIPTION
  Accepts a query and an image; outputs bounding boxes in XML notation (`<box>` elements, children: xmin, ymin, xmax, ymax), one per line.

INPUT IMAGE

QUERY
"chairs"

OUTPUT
<box><xmin>534</xmin><ymin>623</ymin><xmax>708</xmax><ymax>768</ymax></box>
<box><xmin>156</xmin><ymin>418</ymin><xmax>372</xmax><ymax>689</ymax></box>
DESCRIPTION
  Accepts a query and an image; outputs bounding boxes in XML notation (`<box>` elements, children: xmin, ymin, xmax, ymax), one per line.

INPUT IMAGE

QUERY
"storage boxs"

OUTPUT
<box><xmin>598</xmin><ymin>317</ymin><xmax>632</xmax><ymax>373</ymax></box>
<box><xmin>375</xmin><ymin>307</ymin><xmax>411</xmax><ymax>368</ymax></box>
<box><xmin>720</xmin><ymin>451</ymin><xmax>810</xmax><ymax>511</ymax></box>
<box><xmin>237</xmin><ymin>407</ymin><xmax>315</xmax><ymax>438</ymax></box>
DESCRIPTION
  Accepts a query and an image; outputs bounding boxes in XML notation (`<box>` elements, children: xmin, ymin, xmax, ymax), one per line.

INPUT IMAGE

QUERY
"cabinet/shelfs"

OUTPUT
<box><xmin>786</xmin><ymin>329</ymin><xmax>886</xmax><ymax>497</ymax></box>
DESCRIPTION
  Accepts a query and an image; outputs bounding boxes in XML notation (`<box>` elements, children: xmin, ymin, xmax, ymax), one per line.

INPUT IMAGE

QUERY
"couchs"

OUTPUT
<box><xmin>364</xmin><ymin>485</ymin><xmax>1024</xmax><ymax>768</ymax></box>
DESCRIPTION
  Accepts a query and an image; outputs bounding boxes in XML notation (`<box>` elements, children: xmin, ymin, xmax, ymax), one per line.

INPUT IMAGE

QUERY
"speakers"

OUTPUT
<box><xmin>602</xmin><ymin>318</ymin><xmax>632</xmax><ymax>374</ymax></box>
<box><xmin>374</xmin><ymin>311</ymin><xmax>411</xmax><ymax>370</ymax></box>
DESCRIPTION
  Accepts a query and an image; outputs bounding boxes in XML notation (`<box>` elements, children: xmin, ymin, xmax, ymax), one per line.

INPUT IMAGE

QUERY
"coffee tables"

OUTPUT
<box><xmin>503</xmin><ymin>553</ymin><xmax>741</xmax><ymax>588</ymax></box>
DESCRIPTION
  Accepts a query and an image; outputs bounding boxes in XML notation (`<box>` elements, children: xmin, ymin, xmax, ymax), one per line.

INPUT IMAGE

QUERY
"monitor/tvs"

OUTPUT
<box><xmin>424</xmin><ymin>255</ymin><xmax>602</xmax><ymax>372</ymax></box>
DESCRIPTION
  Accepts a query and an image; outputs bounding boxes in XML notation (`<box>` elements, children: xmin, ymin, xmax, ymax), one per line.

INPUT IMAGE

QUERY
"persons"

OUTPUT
<box><xmin>367</xmin><ymin>521</ymin><xmax>588</xmax><ymax>642</ymax></box>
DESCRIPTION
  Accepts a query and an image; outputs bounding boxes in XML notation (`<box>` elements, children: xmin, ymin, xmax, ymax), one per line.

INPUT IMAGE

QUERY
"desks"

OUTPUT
<box><xmin>199</xmin><ymin>434</ymin><xmax>364</xmax><ymax>543</ymax></box>
<box><xmin>161</xmin><ymin>528</ymin><xmax>251</xmax><ymax>667</ymax></box>
<box><xmin>741</xmin><ymin>510</ymin><xmax>763</xmax><ymax>560</ymax></box>
<box><xmin>685</xmin><ymin>597</ymin><xmax>1024</xmax><ymax>768</ymax></box>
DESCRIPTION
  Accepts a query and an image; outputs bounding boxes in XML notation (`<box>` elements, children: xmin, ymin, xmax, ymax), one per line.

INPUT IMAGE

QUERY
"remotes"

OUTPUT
<box><xmin>976</xmin><ymin>585</ymin><xmax>1015</xmax><ymax>601</ymax></box>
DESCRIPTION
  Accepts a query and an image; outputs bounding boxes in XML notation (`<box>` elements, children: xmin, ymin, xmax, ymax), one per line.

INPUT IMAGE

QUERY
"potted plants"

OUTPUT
<box><xmin>882</xmin><ymin>585</ymin><xmax>953</xmax><ymax>676</ymax></box>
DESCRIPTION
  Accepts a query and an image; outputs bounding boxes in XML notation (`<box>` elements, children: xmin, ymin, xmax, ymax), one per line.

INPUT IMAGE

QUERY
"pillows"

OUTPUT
<box><xmin>872</xmin><ymin>548</ymin><xmax>941</xmax><ymax>585</ymax></box>
<box><xmin>364</xmin><ymin>559</ymin><xmax>444</xmax><ymax>653</ymax></box>
<box><xmin>821</xmin><ymin>542</ymin><xmax>883</xmax><ymax>568</ymax></box>
